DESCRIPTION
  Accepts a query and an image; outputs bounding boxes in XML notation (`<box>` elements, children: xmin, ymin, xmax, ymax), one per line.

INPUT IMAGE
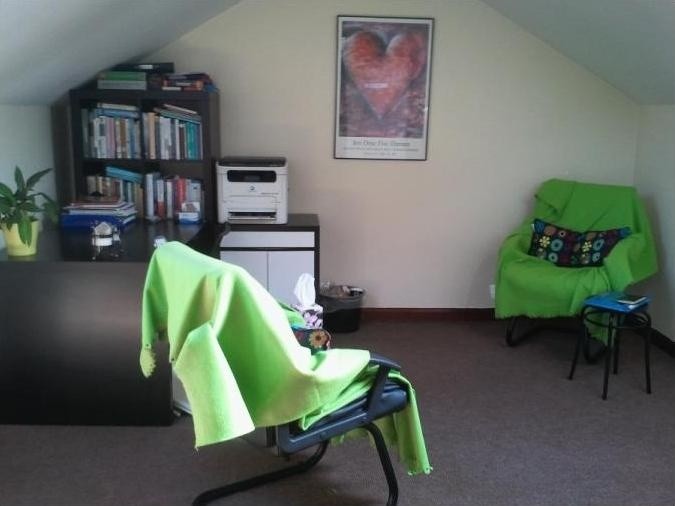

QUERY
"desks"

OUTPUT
<box><xmin>0</xmin><ymin>223</ymin><xmax>204</xmax><ymax>425</ymax></box>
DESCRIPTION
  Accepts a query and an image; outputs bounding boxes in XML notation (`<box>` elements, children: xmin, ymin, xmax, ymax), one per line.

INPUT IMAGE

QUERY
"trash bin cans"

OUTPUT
<box><xmin>319</xmin><ymin>286</ymin><xmax>364</xmax><ymax>333</ymax></box>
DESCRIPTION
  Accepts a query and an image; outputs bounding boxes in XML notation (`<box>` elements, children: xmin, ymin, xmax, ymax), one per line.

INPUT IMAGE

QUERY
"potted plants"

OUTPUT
<box><xmin>1</xmin><ymin>164</ymin><xmax>60</xmax><ymax>257</ymax></box>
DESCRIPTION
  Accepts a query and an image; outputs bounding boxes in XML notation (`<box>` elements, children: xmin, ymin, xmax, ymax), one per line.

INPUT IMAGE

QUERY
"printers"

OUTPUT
<box><xmin>216</xmin><ymin>156</ymin><xmax>286</xmax><ymax>225</ymax></box>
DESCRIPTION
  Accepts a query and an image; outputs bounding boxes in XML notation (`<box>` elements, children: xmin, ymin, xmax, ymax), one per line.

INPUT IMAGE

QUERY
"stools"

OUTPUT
<box><xmin>567</xmin><ymin>297</ymin><xmax>652</xmax><ymax>400</ymax></box>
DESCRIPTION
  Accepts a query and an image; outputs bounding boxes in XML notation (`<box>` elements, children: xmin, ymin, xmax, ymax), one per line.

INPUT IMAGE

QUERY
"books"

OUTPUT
<box><xmin>61</xmin><ymin>62</ymin><xmax>219</xmax><ymax>228</ymax></box>
<box><xmin>614</xmin><ymin>295</ymin><xmax>646</xmax><ymax>304</ymax></box>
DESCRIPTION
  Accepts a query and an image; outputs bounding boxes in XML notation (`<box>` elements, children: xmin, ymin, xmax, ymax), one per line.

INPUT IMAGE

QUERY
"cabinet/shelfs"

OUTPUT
<box><xmin>68</xmin><ymin>87</ymin><xmax>213</xmax><ymax>228</ymax></box>
<box><xmin>219</xmin><ymin>233</ymin><xmax>315</xmax><ymax>313</ymax></box>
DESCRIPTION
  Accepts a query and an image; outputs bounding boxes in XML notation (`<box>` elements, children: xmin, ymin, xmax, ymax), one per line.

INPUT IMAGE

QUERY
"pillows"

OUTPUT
<box><xmin>527</xmin><ymin>218</ymin><xmax>630</xmax><ymax>267</ymax></box>
<box><xmin>291</xmin><ymin>324</ymin><xmax>331</xmax><ymax>352</ymax></box>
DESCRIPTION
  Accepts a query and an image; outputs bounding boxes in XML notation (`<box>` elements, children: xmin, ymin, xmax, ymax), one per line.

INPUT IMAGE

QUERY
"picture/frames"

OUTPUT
<box><xmin>331</xmin><ymin>15</ymin><xmax>434</xmax><ymax>161</ymax></box>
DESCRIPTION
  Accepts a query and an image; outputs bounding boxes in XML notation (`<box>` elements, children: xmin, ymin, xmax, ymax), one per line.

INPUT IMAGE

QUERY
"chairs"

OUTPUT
<box><xmin>499</xmin><ymin>180</ymin><xmax>639</xmax><ymax>361</ymax></box>
<box><xmin>157</xmin><ymin>243</ymin><xmax>408</xmax><ymax>504</ymax></box>
